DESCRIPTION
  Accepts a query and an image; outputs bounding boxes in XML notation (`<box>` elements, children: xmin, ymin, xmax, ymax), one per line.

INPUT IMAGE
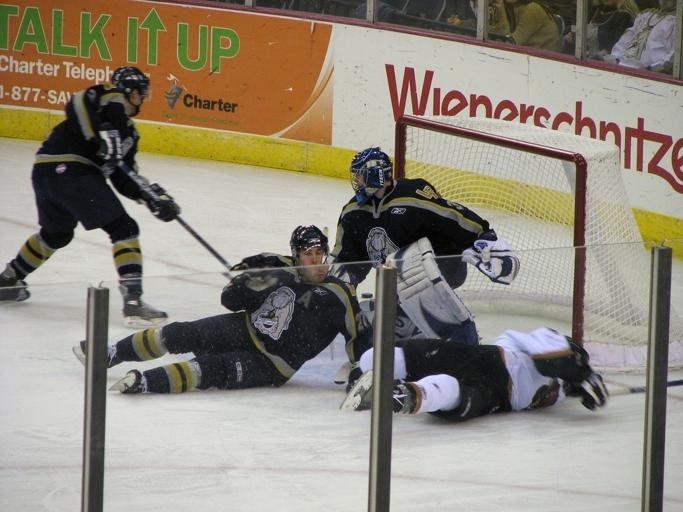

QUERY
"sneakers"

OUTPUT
<box><xmin>81</xmin><ymin>340</ymin><xmax>123</xmax><ymax>367</ymax></box>
<box><xmin>124</xmin><ymin>298</ymin><xmax>167</xmax><ymax>320</ymax></box>
<box><xmin>355</xmin><ymin>383</ymin><xmax>408</xmax><ymax>412</ymax></box>
<box><xmin>122</xmin><ymin>370</ymin><xmax>151</xmax><ymax>393</ymax></box>
<box><xmin>0</xmin><ymin>274</ymin><xmax>30</xmax><ymax>301</ymax></box>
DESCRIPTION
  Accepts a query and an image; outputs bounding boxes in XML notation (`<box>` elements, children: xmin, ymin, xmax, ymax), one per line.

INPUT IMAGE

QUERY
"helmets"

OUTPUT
<box><xmin>290</xmin><ymin>226</ymin><xmax>329</xmax><ymax>266</ymax></box>
<box><xmin>349</xmin><ymin>147</ymin><xmax>393</xmax><ymax>208</ymax></box>
<box><xmin>110</xmin><ymin>67</ymin><xmax>152</xmax><ymax>103</ymax></box>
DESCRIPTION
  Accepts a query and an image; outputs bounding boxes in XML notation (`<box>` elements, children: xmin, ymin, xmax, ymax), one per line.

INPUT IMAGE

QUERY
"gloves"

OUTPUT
<box><xmin>139</xmin><ymin>184</ymin><xmax>180</xmax><ymax>221</ymax></box>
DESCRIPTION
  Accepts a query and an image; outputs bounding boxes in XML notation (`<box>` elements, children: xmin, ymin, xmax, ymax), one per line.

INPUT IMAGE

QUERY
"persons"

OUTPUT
<box><xmin>1</xmin><ymin>65</ymin><xmax>179</xmax><ymax>328</ymax></box>
<box><xmin>340</xmin><ymin>328</ymin><xmax>610</xmax><ymax>422</ymax></box>
<box><xmin>74</xmin><ymin>225</ymin><xmax>368</xmax><ymax>395</ymax></box>
<box><xmin>321</xmin><ymin>151</ymin><xmax>518</xmax><ymax>349</ymax></box>
<box><xmin>255</xmin><ymin>0</ymin><xmax>683</xmax><ymax>78</ymax></box>
